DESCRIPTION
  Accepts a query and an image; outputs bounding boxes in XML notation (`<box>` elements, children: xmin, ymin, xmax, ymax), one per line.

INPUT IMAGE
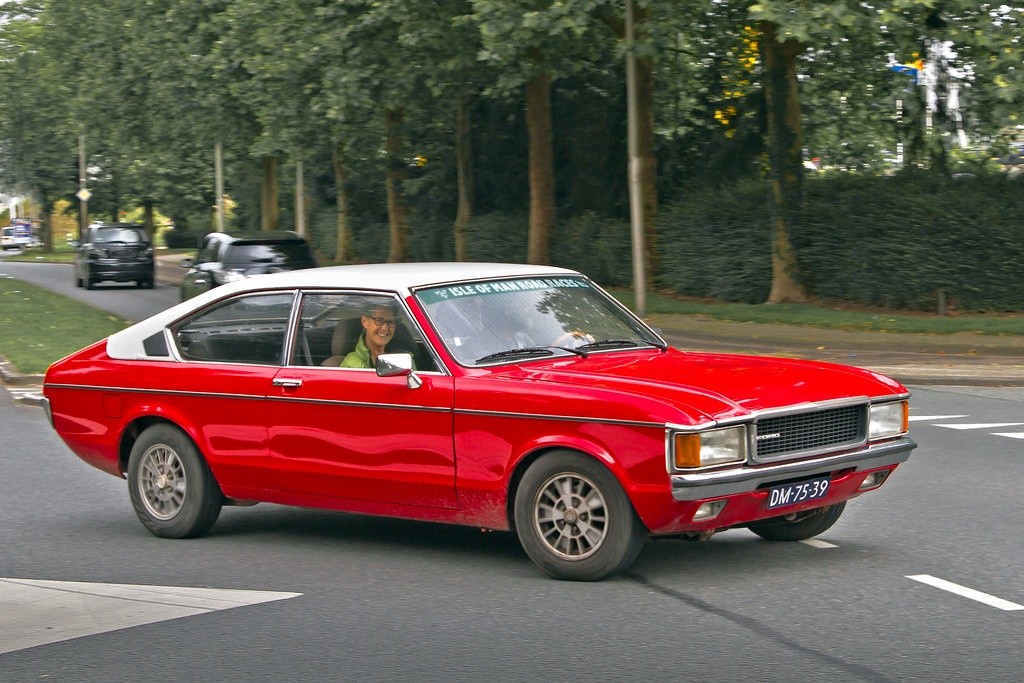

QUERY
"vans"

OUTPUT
<box><xmin>71</xmin><ymin>219</ymin><xmax>154</xmax><ymax>289</ymax></box>
<box><xmin>0</xmin><ymin>226</ymin><xmax>31</xmax><ymax>250</ymax></box>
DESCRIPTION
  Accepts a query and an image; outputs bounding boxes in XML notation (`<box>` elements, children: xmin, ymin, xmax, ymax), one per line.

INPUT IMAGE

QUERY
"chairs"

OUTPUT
<box><xmin>321</xmin><ymin>316</ymin><xmax>418</xmax><ymax>369</ymax></box>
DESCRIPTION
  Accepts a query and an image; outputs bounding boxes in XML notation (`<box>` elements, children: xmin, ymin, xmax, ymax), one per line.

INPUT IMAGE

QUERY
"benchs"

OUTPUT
<box><xmin>191</xmin><ymin>324</ymin><xmax>333</xmax><ymax>365</ymax></box>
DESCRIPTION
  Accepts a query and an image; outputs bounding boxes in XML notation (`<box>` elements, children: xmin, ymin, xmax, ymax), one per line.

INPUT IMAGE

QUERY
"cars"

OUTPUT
<box><xmin>38</xmin><ymin>262</ymin><xmax>917</xmax><ymax>582</ymax></box>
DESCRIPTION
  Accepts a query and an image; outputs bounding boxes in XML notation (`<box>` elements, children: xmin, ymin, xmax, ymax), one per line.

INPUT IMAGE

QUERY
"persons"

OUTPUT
<box><xmin>466</xmin><ymin>294</ymin><xmax>540</xmax><ymax>355</ymax></box>
<box><xmin>340</xmin><ymin>300</ymin><xmax>400</xmax><ymax>372</ymax></box>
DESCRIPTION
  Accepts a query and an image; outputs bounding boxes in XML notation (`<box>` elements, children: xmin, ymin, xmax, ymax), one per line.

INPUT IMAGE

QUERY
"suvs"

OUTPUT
<box><xmin>180</xmin><ymin>229</ymin><xmax>318</xmax><ymax>306</ymax></box>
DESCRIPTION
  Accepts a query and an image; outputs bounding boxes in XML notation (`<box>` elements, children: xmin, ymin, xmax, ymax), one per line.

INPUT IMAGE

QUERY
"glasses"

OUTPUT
<box><xmin>368</xmin><ymin>315</ymin><xmax>397</xmax><ymax>328</ymax></box>
